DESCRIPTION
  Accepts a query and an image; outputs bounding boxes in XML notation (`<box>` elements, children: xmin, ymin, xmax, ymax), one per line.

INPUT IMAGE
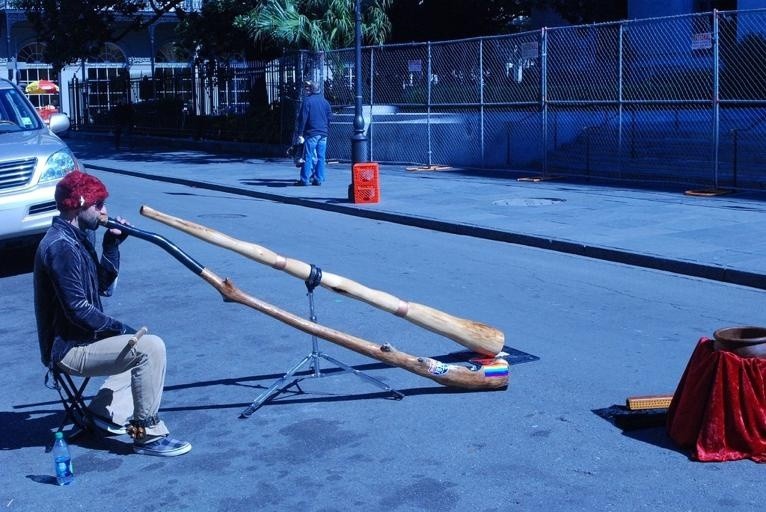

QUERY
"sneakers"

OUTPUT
<box><xmin>293</xmin><ymin>177</ymin><xmax>322</xmax><ymax>187</ymax></box>
<box><xmin>82</xmin><ymin>407</ymin><xmax>128</xmax><ymax>435</ymax></box>
<box><xmin>132</xmin><ymin>435</ymin><xmax>192</xmax><ymax>457</ymax></box>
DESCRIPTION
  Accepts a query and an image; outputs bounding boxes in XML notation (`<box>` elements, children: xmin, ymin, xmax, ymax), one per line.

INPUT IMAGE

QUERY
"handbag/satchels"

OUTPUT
<box><xmin>287</xmin><ymin>142</ymin><xmax>318</xmax><ymax>168</ymax></box>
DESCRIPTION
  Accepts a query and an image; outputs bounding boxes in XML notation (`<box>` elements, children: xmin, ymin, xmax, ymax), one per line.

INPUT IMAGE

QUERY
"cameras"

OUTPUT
<box><xmin>286</xmin><ymin>144</ymin><xmax>305</xmax><ymax>168</ymax></box>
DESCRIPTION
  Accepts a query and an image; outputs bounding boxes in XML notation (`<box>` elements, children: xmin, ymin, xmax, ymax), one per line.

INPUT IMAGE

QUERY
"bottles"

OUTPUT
<box><xmin>52</xmin><ymin>433</ymin><xmax>74</xmax><ymax>487</ymax></box>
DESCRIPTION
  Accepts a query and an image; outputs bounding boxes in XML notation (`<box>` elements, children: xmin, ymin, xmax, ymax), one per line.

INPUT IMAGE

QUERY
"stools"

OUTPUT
<box><xmin>47</xmin><ymin>372</ymin><xmax>106</xmax><ymax>449</ymax></box>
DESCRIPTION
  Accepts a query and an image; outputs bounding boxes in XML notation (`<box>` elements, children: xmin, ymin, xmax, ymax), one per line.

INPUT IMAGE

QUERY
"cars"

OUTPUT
<box><xmin>0</xmin><ymin>77</ymin><xmax>96</xmax><ymax>250</ymax></box>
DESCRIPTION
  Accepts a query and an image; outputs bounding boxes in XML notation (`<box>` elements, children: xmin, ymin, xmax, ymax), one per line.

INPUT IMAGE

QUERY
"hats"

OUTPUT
<box><xmin>305</xmin><ymin>81</ymin><xmax>321</xmax><ymax>95</ymax></box>
<box><xmin>54</xmin><ymin>170</ymin><xmax>109</xmax><ymax>212</ymax></box>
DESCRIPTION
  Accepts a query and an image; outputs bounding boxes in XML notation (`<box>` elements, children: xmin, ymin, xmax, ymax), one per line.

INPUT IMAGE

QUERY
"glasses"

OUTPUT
<box><xmin>92</xmin><ymin>201</ymin><xmax>106</xmax><ymax>208</ymax></box>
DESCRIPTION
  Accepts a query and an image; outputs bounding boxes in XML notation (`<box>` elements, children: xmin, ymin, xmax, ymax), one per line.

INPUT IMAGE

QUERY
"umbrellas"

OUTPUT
<box><xmin>23</xmin><ymin>78</ymin><xmax>60</xmax><ymax>108</ymax></box>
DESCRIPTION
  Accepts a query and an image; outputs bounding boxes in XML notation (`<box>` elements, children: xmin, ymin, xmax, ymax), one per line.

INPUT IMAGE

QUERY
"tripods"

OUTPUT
<box><xmin>240</xmin><ymin>291</ymin><xmax>405</xmax><ymax>420</ymax></box>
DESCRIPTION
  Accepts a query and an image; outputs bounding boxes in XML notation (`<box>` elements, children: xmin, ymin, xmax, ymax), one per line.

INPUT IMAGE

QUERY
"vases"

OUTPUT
<box><xmin>712</xmin><ymin>328</ymin><xmax>765</xmax><ymax>357</ymax></box>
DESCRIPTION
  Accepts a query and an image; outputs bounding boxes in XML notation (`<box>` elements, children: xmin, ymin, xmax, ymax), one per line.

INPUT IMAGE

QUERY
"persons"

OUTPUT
<box><xmin>293</xmin><ymin>81</ymin><xmax>332</xmax><ymax>186</ymax></box>
<box><xmin>293</xmin><ymin>80</ymin><xmax>318</xmax><ymax>179</ymax></box>
<box><xmin>31</xmin><ymin>169</ymin><xmax>192</xmax><ymax>457</ymax></box>
